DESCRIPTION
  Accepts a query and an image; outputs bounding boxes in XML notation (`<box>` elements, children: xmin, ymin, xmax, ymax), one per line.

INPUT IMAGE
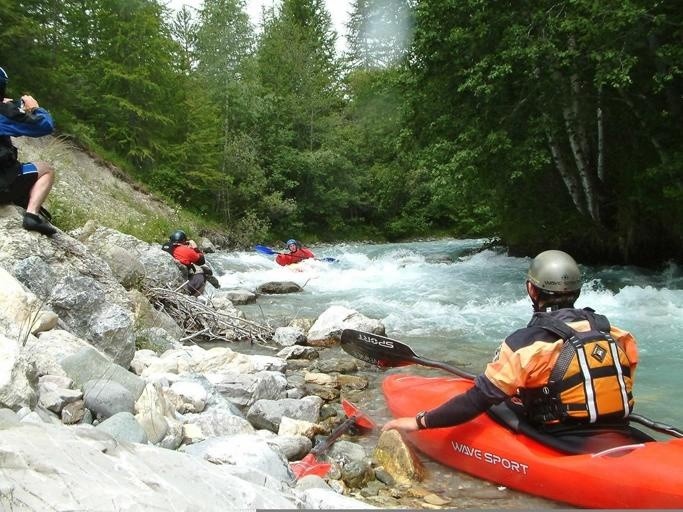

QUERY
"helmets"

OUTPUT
<box><xmin>287</xmin><ymin>239</ymin><xmax>296</xmax><ymax>247</ymax></box>
<box><xmin>171</xmin><ymin>230</ymin><xmax>186</xmax><ymax>241</ymax></box>
<box><xmin>0</xmin><ymin>67</ymin><xmax>8</xmax><ymax>103</ymax></box>
<box><xmin>527</xmin><ymin>249</ymin><xmax>582</xmax><ymax>293</ymax></box>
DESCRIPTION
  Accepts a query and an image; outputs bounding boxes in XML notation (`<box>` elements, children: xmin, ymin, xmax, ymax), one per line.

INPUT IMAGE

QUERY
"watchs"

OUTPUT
<box><xmin>415</xmin><ymin>410</ymin><xmax>427</xmax><ymax>431</ymax></box>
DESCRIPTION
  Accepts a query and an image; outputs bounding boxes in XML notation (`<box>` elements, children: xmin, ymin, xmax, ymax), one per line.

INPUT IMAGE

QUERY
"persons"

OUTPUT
<box><xmin>378</xmin><ymin>248</ymin><xmax>637</xmax><ymax>449</ymax></box>
<box><xmin>0</xmin><ymin>69</ymin><xmax>59</xmax><ymax>236</ymax></box>
<box><xmin>161</xmin><ymin>231</ymin><xmax>208</xmax><ymax>297</ymax></box>
<box><xmin>275</xmin><ymin>239</ymin><xmax>315</xmax><ymax>267</ymax></box>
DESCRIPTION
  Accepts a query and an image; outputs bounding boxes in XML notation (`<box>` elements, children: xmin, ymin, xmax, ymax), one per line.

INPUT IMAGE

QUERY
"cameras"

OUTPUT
<box><xmin>10</xmin><ymin>100</ymin><xmax>24</xmax><ymax>109</ymax></box>
<box><xmin>184</xmin><ymin>242</ymin><xmax>190</xmax><ymax>245</ymax></box>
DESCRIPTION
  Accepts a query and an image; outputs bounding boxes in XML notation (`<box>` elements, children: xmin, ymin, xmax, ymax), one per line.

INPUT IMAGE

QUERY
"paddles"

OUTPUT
<box><xmin>288</xmin><ymin>399</ymin><xmax>373</xmax><ymax>482</ymax></box>
<box><xmin>340</xmin><ymin>330</ymin><xmax>683</xmax><ymax>439</ymax></box>
<box><xmin>256</xmin><ymin>246</ymin><xmax>340</xmax><ymax>264</ymax></box>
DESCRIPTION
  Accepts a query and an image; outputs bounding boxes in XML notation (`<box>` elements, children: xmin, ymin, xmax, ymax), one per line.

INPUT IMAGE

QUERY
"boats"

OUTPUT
<box><xmin>382</xmin><ymin>372</ymin><xmax>683</xmax><ymax>511</ymax></box>
<box><xmin>276</xmin><ymin>249</ymin><xmax>315</xmax><ymax>267</ymax></box>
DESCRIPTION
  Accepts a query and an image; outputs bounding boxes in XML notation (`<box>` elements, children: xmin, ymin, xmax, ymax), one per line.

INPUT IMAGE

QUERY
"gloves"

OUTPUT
<box><xmin>189</xmin><ymin>240</ymin><xmax>198</xmax><ymax>249</ymax></box>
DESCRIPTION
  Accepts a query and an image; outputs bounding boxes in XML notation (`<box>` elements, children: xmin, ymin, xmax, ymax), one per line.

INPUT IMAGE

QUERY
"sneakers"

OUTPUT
<box><xmin>38</xmin><ymin>206</ymin><xmax>53</xmax><ymax>221</ymax></box>
<box><xmin>23</xmin><ymin>213</ymin><xmax>57</xmax><ymax>235</ymax></box>
